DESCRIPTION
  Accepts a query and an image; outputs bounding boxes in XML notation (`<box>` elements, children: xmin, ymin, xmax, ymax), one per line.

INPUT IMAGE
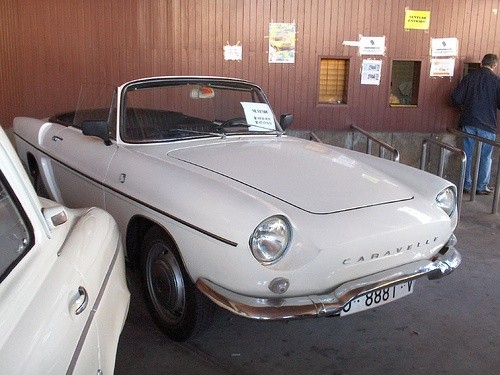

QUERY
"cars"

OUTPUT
<box><xmin>13</xmin><ymin>72</ymin><xmax>461</xmax><ymax>342</ymax></box>
<box><xmin>0</xmin><ymin>123</ymin><xmax>131</xmax><ymax>375</ymax></box>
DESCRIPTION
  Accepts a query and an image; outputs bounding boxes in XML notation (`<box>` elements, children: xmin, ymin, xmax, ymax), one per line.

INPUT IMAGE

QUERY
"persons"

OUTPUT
<box><xmin>451</xmin><ymin>53</ymin><xmax>500</xmax><ymax>194</ymax></box>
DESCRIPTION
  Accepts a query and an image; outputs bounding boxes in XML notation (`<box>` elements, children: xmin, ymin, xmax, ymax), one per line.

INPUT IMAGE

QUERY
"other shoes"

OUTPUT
<box><xmin>476</xmin><ymin>186</ymin><xmax>494</xmax><ymax>195</ymax></box>
<box><xmin>462</xmin><ymin>186</ymin><xmax>472</xmax><ymax>193</ymax></box>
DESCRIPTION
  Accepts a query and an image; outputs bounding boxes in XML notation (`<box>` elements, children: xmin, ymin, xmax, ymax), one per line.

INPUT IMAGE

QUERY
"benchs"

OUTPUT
<box><xmin>53</xmin><ymin>108</ymin><xmax>216</xmax><ymax>141</ymax></box>
